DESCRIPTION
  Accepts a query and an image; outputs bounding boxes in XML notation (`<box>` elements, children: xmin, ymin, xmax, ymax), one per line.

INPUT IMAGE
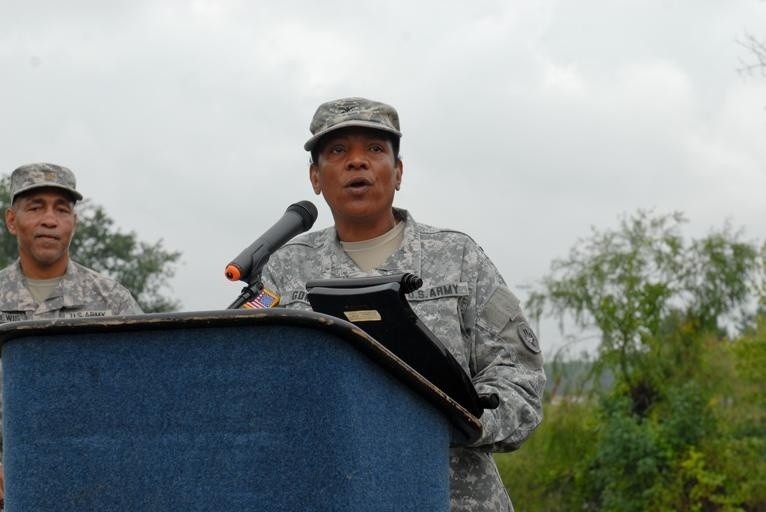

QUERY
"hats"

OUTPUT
<box><xmin>11</xmin><ymin>163</ymin><xmax>83</xmax><ymax>207</ymax></box>
<box><xmin>304</xmin><ymin>97</ymin><xmax>402</xmax><ymax>151</ymax></box>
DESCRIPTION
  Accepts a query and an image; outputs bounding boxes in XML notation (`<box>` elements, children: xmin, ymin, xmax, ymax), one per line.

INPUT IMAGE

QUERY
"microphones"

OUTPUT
<box><xmin>225</xmin><ymin>201</ymin><xmax>317</xmax><ymax>281</ymax></box>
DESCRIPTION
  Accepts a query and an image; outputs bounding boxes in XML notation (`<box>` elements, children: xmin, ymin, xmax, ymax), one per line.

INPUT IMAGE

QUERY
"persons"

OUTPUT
<box><xmin>237</xmin><ymin>97</ymin><xmax>546</xmax><ymax>512</ymax></box>
<box><xmin>0</xmin><ymin>162</ymin><xmax>147</xmax><ymax>325</ymax></box>
